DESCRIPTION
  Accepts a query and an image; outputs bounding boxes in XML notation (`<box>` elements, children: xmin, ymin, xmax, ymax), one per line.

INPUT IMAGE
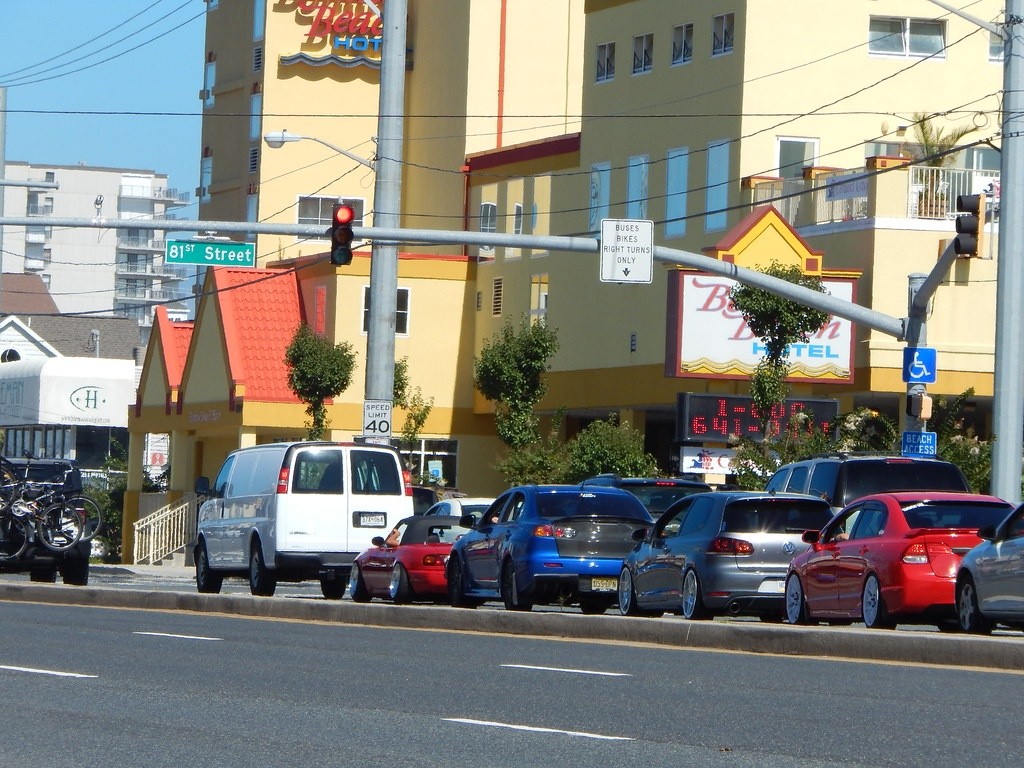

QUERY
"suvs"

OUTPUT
<box><xmin>2</xmin><ymin>455</ymin><xmax>94</xmax><ymax>586</ymax></box>
<box><xmin>554</xmin><ymin>472</ymin><xmax>714</xmax><ymax>537</ymax></box>
<box><xmin>762</xmin><ymin>451</ymin><xmax>975</xmax><ymax>539</ymax></box>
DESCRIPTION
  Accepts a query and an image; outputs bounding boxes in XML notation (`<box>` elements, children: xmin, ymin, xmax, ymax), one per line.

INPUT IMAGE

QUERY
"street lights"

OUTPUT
<box><xmin>262</xmin><ymin>127</ymin><xmax>401</xmax><ymax>445</ymax></box>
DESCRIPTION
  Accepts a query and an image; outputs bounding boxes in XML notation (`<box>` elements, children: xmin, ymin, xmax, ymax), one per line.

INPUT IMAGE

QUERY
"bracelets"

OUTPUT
<box><xmin>833</xmin><ymin>535</ymin><xmax>837</xmax><ymax>541</ymax></box>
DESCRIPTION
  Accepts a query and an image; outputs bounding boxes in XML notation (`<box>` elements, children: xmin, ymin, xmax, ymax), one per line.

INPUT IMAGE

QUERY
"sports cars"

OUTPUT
<box><xmin>349</xmin><ymin>514</ymin><xmax>482</xmax><ymax>606</ymax></box>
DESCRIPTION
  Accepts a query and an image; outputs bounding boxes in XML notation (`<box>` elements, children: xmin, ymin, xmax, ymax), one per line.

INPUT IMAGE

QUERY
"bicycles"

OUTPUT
<box><xmin>0</xmin><ymin>448</ymin><xmax>103</xmax><ymax>562</ymax></box>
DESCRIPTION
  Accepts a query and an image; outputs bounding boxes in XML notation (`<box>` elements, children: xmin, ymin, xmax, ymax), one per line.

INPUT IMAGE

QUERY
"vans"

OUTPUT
<box><xmin>190</xmin><ymin>440</ymin><xmax>415</xmax><ymax>600</ymax></box>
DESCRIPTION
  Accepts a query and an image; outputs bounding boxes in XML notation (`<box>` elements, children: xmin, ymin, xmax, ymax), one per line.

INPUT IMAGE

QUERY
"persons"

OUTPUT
<box><xmin>829</xmin><ymin>534</ymin><xmax>849</xmax><ymax>543</ymax></box>
<box><xmin>385</xmin><ymin>528</ymin><xmax>402</xmax><ymax>546</ymax></box>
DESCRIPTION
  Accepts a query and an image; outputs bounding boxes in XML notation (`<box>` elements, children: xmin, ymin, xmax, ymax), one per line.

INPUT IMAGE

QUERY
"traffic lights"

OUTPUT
<box><xmin>954</xmin><ymin>192</ymin><xmax>986</xmax><ymax>259</ymax></box>
<box><xmin>329</xmin><ymin>204</ymin><xmax>353</xmax><ymax>266</ymax></box>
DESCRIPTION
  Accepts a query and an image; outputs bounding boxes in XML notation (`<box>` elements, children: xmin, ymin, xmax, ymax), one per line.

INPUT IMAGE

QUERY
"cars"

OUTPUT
<box><xmin>424</xmin><ymin>497</ymin><xmax>525</xmax><ymax>547</ymax></box>
<box><xmin>784</xmin><ymin>491</ymin><xmax>1023</xmax><ymax>633</ymax></box>
<box><xmin>411</xmin><ymin>483</ymin><xmax>469</xmax><ymax>515</ymax></box>
<box><xmin>953</xmin><ymin>500</ymin><xmax>1024</xmax><ymax>636</ymax></box>
<box><xmin>445</xmin><ymin>483</ymin><xmax>657</xmax><ymax>615</ymax></box>
<box><xmin>616</xmin><ymin>490</ymin><xmax>845</xmax><ymax>624</ymax></box>
